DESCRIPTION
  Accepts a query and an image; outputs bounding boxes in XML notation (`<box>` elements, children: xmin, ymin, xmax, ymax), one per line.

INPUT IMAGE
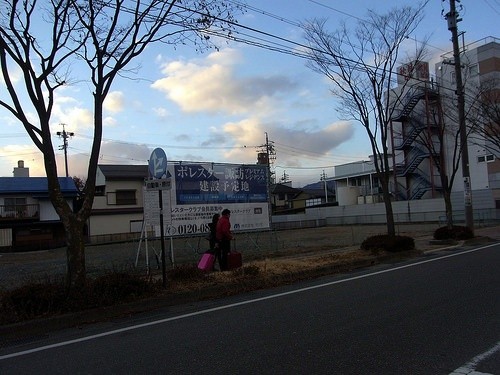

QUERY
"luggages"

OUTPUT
<box><xmin>226</xmin><ymin>238</ymin><xmax>241</xmax><ymax>270</ymax></box>
<box><xmin>198</xmin><ymin>247</ymin><xmax>216</xmax><ymax>274</ymax></box>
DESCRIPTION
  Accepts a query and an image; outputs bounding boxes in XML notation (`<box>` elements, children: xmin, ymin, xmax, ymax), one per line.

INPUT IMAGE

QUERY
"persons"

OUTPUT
<box><xmin>214</xmin><ymin>209</ymin><xmax>235</xmax><ymax>271</ymax></box>
<box><xmin>204</xmin><ymin>213</ymin><xmax>223</xmax><ymax>268</ymax></box>
